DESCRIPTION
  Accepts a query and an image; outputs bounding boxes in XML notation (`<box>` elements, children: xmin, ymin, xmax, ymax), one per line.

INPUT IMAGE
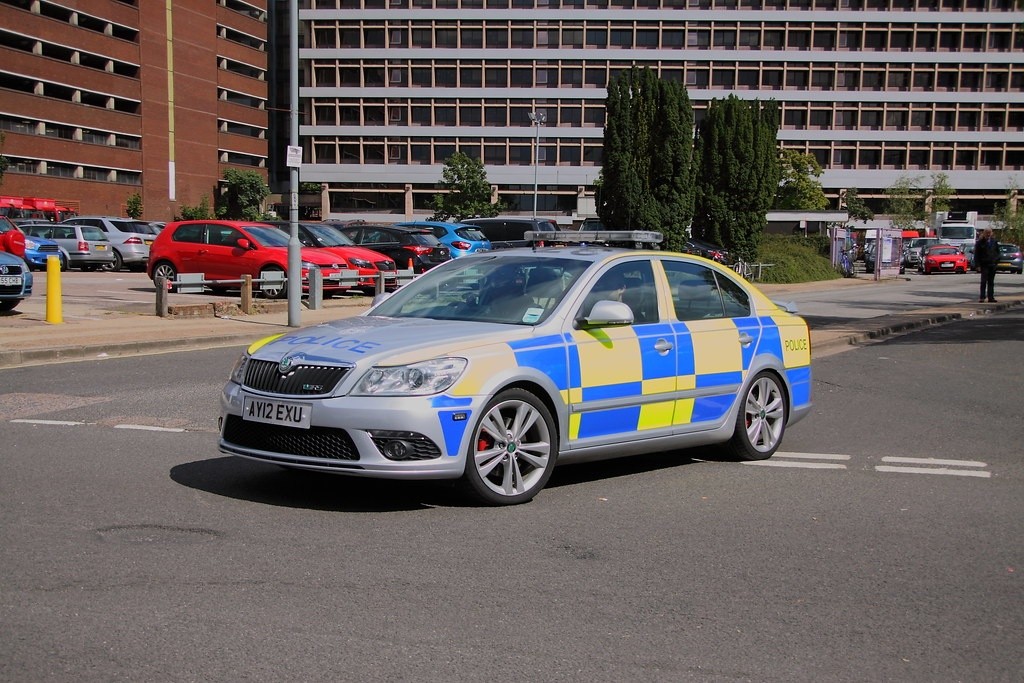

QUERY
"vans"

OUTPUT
<box><xmin>459</xmin><ymin>215</ymin><xmax>568</xmax><ymax>251</ymax></box>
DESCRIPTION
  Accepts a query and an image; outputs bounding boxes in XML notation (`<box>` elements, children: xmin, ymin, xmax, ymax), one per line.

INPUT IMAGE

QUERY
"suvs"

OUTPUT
<box><xmin>318</xmin><ymin>217</ymin><xmax>452</xmax><ymax>274</ymax></box>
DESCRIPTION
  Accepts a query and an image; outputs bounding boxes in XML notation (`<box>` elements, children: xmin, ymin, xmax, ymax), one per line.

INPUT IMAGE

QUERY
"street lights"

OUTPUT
<box><xmin>527</xmin><ymin>112</ymin><xmax>547</xmax><ymax>218</ymax></box>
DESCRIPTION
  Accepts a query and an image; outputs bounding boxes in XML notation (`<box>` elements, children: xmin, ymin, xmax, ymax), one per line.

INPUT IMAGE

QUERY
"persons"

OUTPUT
<box><xmin>589</xmin><ymin>268</ymin><xmax>646</xmax><ymax>323</ymax></box>
<box><xmin>974</xmin><ymin>227</ymin><xmax>1001</xmax><ymax>302</ymax></box>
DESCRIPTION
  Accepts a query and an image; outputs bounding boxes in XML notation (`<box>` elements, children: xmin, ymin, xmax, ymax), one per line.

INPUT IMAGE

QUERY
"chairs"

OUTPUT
<box><xmin>678</xmin><ymin>281</ymin><xmax>711</xmax><ymax>320</ymax></box>
<box><xmin>624</xmin><ymin>278</ymin><xmax>645</xmax><ymax>324</ymax></box>
<box><xmin>32</xmin><ymin>231</ymin><xmax>76</xmax><ymax>239</ymax></box>
<box><xmin>524</xmin><ymin>268</ymin><xmax>563</xmax><ymax>313</ymax></box>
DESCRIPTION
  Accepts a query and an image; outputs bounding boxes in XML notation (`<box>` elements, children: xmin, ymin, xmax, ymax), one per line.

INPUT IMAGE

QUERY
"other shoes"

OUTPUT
<box><xmin>979</xmin><ymin>297</ymin><xmax>986</xmax><ymax>302</ymax></box>
<box><xmin>988</xmin><ymin>297</ymin><xmax>997</xmax><ymax>303</ymax></box>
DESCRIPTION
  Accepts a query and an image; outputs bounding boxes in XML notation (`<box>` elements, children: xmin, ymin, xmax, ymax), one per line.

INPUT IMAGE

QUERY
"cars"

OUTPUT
<box><xmin>145</xmin><ymin>219</ymin><xmax>359</xmax><ymax>300</ymax></box>
<box><xmin>862</xmin><ymin>236</ymin><xmax>1023</xmax><ymax>274</ymax></box>
<box><xmin>0</xmin><ymin>250</ymin><xmax>34</xmax><ymax>311</ymax></box>
<box><xmin>0</xmin><ymin>196</ymin><xmax>169</xmax><ymax>273</ymax></box>
<box><xmin>571</xmin><ymin>217</ymin><xmax>611</xmax><ymax>246</ymax></box>
<box><xmin>217</xmin><ymin>228</ymin><xmax>813</xmax><ymax>507</ymax></box>
<box><xmin>392</xmin><ymin>221</ymin><xmax>492</xmax><ymax>261</ymax></box>
<box><xmin>253</xmin><ymin>219</ymin><xmax>398</xmax><ymax>295</ymax></box>
